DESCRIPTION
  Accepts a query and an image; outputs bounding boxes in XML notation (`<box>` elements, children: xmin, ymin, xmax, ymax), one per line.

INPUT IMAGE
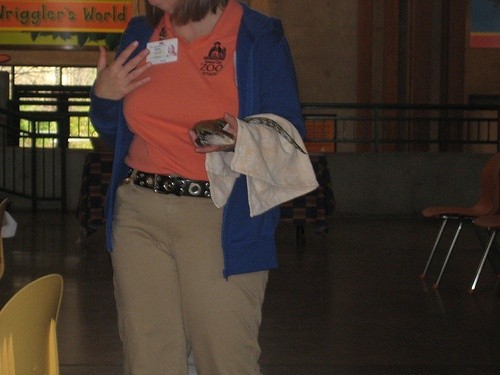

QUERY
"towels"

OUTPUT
<box><xmin>199</xmin><ymin>114</ymin><xmax>321</xmax><ymax>221</ymax></box>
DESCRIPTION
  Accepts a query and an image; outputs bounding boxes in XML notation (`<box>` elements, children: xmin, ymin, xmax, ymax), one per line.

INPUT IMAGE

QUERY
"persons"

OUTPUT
<box><xmin>91</xmin><ymin>0</ymin><xmax>305</xmax><ymax>374</ymax></box>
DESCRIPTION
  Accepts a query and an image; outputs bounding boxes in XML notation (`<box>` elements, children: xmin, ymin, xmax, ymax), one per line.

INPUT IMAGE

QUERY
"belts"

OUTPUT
<box><xmin>122</xmin><ymin>163</ymin><xmax>212</xmax><ymax>198</ymax></box>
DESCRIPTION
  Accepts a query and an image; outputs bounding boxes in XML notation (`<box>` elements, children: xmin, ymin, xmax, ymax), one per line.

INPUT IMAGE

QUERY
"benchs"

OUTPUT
<box><xmin>75</xmin><ymin>150</ymin><xmax>336</xmax><ymax>249</ymax></box>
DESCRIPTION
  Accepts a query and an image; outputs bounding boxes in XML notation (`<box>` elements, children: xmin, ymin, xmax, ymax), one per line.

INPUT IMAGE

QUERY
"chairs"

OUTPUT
<box><xmin>0</xmin><ymin>196</ymin><xmax>64</xmax><ymax>375</ymax></box>
<box><xmin>420</xmin><ymin>153</ymin><xmax>500</xmax><ymax>291</ymax></box>
